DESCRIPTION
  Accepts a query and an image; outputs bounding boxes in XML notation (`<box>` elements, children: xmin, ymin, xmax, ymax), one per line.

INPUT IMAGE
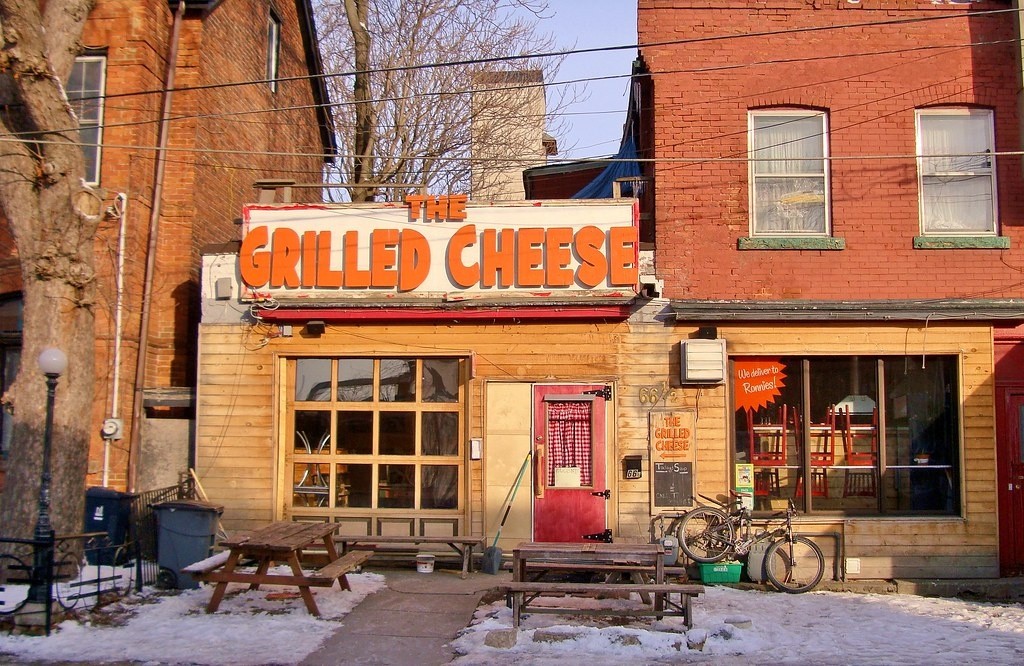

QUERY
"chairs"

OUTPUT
<box><xmin>745</xmin><ymin>404</ymin><xmax>881</xmax><ymax>500</ymax></box>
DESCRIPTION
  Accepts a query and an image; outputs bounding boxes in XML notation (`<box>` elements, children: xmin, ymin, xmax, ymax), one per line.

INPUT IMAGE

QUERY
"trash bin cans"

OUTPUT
<box><xmin>85</xmin><ymin>487</ymin><xmax>141</xmax><ymax>566</ymax></box>
<box><xmin>148</xmin><ymin>500</ymin><xmax>223</xmax><ymax>591</ymax></box>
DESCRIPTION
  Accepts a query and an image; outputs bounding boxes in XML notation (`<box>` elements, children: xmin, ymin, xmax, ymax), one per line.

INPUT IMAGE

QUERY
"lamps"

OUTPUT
<box><xmin>40</xmin><ymin>346</ymin><xmax>68</xmax><ymax>390</ymax></box>
<box><xmin>306</xmin><ymin>319</ymin><xmax>327</xmax><ymax>336</ymax></box>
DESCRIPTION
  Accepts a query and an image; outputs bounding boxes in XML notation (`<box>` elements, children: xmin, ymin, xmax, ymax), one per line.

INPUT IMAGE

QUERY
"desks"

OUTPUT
<box><xmin>752</xmin><ymin>465</ymin><xmax>963</xmax><ymax>513</ymax></box>
<box><xmin>209</xmin><ymin>523</ymin><xmax>352</xmax><ymax>617</ymax></box>
<box><xmin>510</xmin><ymin>541</ymin><xmax>665</xmax><ymax>629</ymax></box>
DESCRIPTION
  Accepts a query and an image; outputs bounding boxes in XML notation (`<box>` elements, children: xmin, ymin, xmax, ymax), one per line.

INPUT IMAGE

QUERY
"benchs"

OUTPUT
<box><xmin>499</xmin><ymin>561</ymin><xmax>706</xmax><ymax>629</ymax></box>
<box><xmin>330</xmin><ymin>533</ymin><xmax>486</xmax><ymax>579</ymax></box>
<box><xmin>181</xmin><ymin>549</ymin><xmax>376</xmax><ymax>587</ymax></box>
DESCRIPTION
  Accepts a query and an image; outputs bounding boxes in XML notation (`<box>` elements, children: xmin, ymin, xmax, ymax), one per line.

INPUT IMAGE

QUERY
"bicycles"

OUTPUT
<box><xmin>678</xmin><ymin>489</ymin><xmax>825</xmax><ymax>595</ymax></box>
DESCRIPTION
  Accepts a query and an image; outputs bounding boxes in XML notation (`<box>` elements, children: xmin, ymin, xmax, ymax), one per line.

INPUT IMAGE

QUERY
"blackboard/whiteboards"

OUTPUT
<box><xmin>654</xmin><ymin>461</ymin><xmax>693</xmax><ymax>508</ymax></box>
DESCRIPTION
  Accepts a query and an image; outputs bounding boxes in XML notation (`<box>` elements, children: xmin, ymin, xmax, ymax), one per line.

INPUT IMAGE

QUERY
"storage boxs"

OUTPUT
<box><xmin>696</xmin><ymin>561</ymin><xmax>745</xmax><ymax>584</ymax></box>
